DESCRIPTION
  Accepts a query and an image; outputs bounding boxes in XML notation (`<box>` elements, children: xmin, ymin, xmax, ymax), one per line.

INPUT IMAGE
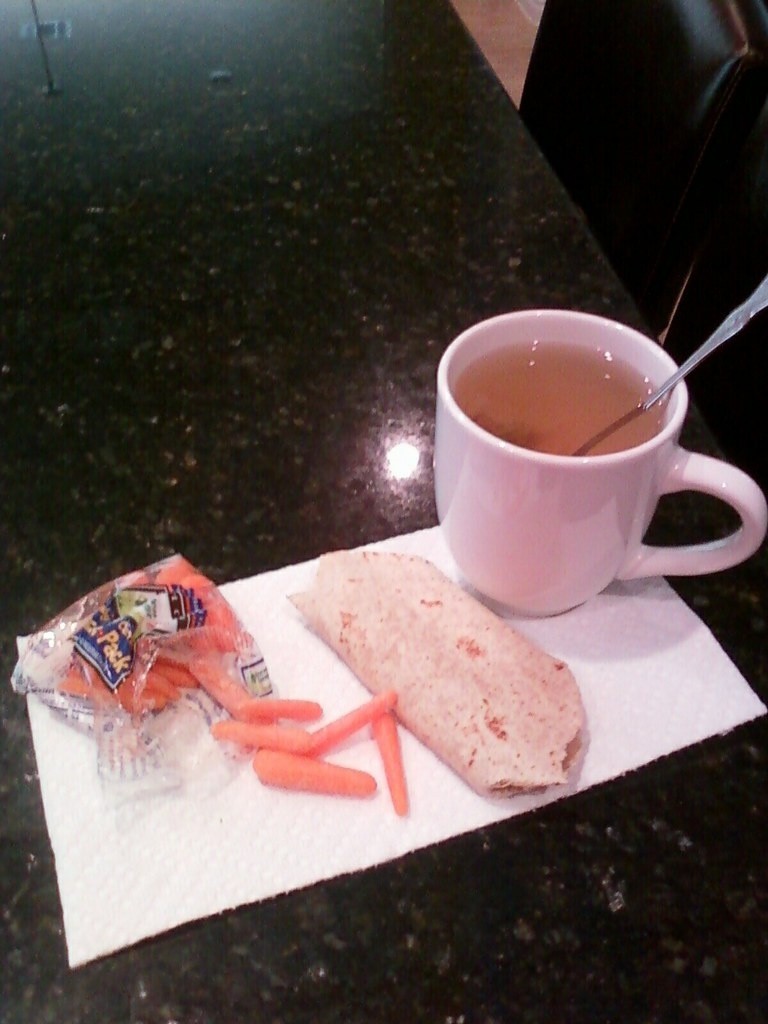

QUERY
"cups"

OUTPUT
<box><xmin>433</xmin><ymin>311</ymin><xmax>767</xmax><ymax>618</ymax></box>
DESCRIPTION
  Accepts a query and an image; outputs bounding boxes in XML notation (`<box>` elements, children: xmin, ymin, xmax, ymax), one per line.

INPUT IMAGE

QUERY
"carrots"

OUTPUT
<box><xmin>54</xmin><ymin>559</ymin><xmax>409</xmax><ymax>819</ymax></box>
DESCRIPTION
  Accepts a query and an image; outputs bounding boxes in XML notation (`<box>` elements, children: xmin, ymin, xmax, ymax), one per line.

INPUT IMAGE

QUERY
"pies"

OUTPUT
<box><xmin>288</xmin><ymin>549</ymin><xmax>583</xmax><ymax>799</ymax></box>
